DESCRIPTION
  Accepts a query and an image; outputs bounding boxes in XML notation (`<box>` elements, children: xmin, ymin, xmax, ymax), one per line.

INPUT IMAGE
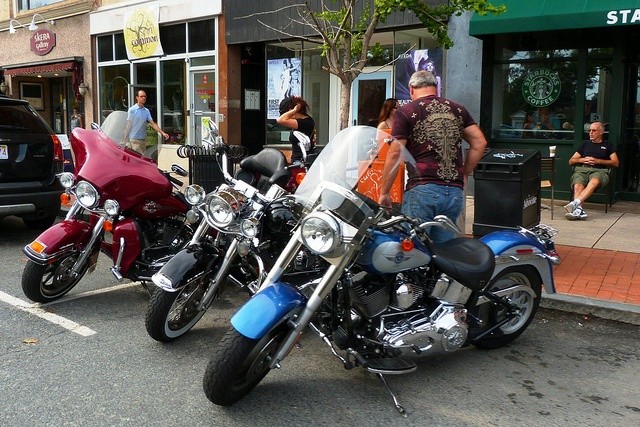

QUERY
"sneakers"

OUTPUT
<box><xmin>564</xmin><ymin>202</ymin><xmax>576</xmax><ymax>212</ymax></box>
<box><xmin>565</xmin><ymin>208</ymin><xmax>588</xmax><ymax>220</ymax></box>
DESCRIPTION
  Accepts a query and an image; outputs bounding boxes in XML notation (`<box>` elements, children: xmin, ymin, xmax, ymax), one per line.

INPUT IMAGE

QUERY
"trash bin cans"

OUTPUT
<box><xmin>473</xmin><ymin>149</ymin><xmax>541</xmax><ymax>239</ymax></box>
<box><xmin>173</xmin><ymin>145</ymin><xmax>246</xmax><ymax>190</ymax></box>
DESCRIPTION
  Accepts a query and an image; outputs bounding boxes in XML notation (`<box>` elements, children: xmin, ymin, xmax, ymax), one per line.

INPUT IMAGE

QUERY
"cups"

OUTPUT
<box><xmin>550</xmin><ymin>146</ymin><xmax>557</xmax><ymax>158</ymax></box>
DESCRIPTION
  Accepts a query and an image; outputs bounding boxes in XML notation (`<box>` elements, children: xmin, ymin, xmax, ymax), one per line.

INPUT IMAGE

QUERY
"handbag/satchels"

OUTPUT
<box><xmin>119</xmin><ymin>139</ymin><xmax>131</xmax><ymax>149</ymax></box>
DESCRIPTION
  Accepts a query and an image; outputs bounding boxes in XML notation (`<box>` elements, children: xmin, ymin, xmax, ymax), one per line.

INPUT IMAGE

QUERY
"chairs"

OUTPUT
<box><xmin>569</xmin><ymin>153</ymin><xmax>615</xmax><ymax>212</ymax></box>
<box><xmin>538</xmin><ymin>155</ymin><xmax>557</xmax><ymax>221</ymax></box>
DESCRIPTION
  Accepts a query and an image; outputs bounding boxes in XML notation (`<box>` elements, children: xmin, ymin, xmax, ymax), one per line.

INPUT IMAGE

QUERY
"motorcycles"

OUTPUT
<box><xmin>20</xmin><ymin>110</ymin><xmax>201</xmax><ymax>305</ymax></box>
<box><xmin>144</xmin><ymin>120</ymin><xmax>329</xmax><ymax>344</ymax></box>
<box><xmin>201</xmin><ymin>124</ymin><xmax>562</xmax><ymax>416</ymax></box>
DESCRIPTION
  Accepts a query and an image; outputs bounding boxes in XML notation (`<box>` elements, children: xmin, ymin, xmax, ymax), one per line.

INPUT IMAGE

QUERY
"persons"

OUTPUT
<box><xmin>521</xmin><ymin>110</ymin><xmax>535</xmax><ymax>138</ymax></box>
<box><xmin>377</xmin><ymin>97</ymin><xmax>405</xmax><ymax>160</ymax></box>
<box><xmin>124</xmin><ymin>89</ymin><xmax>170</xmax><ymax>155</ymax></box>
<box><xmin>377</xmin><ymin>70</ymin><xmax>488</xmax><ymax>243</ymax></box>
<box><xmin>563</xmin><ymin>120</ymin><xmax>619</xmax><ymax>221</ymax></box>
<box><xmin>277</xmin><ymin>96</ymin><xmax>315</xmax><ymax>162</ymax></box>
<box><xmin>70</xmin><ymin>107</ymin><xmax>84</xmax><ymax>132</ymax></box>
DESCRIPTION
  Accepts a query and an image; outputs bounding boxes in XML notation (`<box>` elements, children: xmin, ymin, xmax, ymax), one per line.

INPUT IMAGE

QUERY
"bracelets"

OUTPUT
<box><xmin>289</xmin><ymin>110</ymin><xmax>297</xmax><ymax>117</ymax></box>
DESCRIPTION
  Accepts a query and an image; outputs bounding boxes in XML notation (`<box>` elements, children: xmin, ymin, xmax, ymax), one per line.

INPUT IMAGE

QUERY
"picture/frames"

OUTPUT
<box><xmin>19</xmin><ymin>81</ymin><xmax>45</xmax><ymax>111</ymax></box>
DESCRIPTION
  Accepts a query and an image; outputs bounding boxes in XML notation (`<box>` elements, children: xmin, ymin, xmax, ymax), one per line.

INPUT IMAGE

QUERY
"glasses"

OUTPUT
<box><xmin>588</xmin><ymin>129</ymin><xmax>596</xmax><ymax>131</ymax></box>
<box><xmin>394</xmin><ymin>107</ymin><xmax>398</xmax><ymax>109</ymax></box>
<box><xmin>140</xmin><ymin>96</ymin><xmax>146</xmax><ymax>97</ymax></box>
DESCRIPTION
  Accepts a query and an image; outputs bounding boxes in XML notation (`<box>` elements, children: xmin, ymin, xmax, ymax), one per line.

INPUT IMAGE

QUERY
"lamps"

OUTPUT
<box><xmin>78</xmin><ymin>83</ymin><xmax>89</xmax><ymax>96</ymax></box>
<box><xmin>27</xmin><ymin>13</ymin><xmax>44</xmax><ymax>30</ymax></box>
<box><xmin>7</xmin><ymin>17</ymin><xmax>19</xmax><ymax>33</ymax></box>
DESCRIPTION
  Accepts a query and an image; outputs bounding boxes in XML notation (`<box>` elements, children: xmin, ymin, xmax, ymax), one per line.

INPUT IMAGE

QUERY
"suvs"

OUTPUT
<box><xmin>0</xmin><ymin>98</ymin><xmax>70</xmax><ymax>231</ymax></box>
